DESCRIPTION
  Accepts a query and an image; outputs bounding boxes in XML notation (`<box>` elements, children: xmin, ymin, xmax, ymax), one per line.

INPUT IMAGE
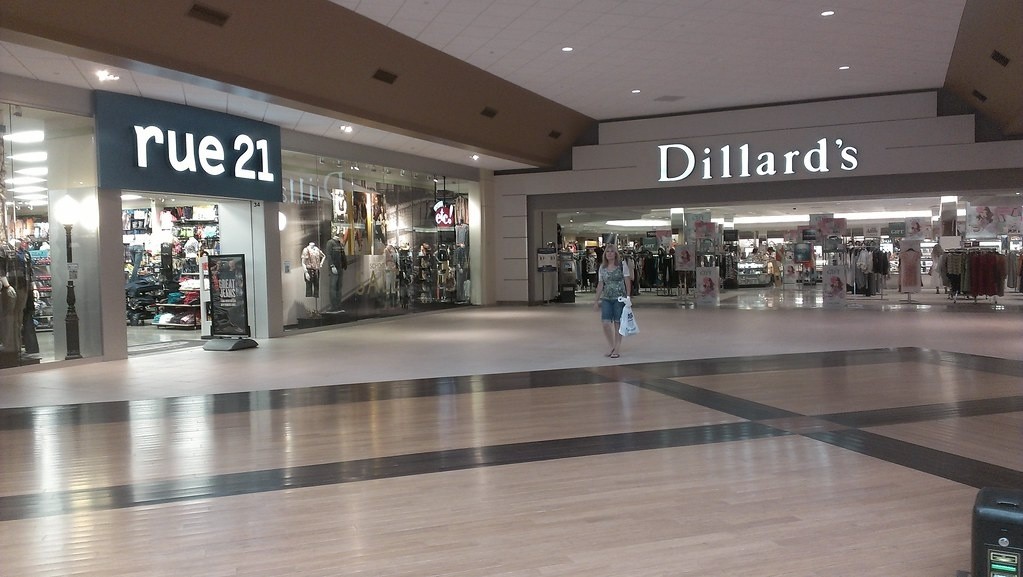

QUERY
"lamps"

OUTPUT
<box><xmin>350</xmin><ymin>162</ymin><xmax>359</xmax><ymax>171</ymax></box>
<box><xmin>384</xmin><ymin>167</ymin><xmax>392</xmax><ymax>174</ymax></box>
<box><xmin>400</xmin><ymin>169</ymin><xmax>406</xmax><ymax>177</ymax></box>
<box><xmin>434</xmin><ymin>175</ymin><xmax>440</xmax><ymax>183</ymax></box>
<box><xmin>370</xmin><ymin>164</ymin><xmax>377</xmax><ymax>172</ymax></box>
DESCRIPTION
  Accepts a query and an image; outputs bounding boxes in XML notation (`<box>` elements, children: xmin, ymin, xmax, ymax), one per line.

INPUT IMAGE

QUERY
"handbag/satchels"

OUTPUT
<box><xmin>618</xmin><ymin>297</ymin><xmax>639</xmax><ymax>336</ymax></box>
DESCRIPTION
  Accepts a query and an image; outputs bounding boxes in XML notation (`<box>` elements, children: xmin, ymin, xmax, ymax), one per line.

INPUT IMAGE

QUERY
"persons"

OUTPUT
<box><xmin>211</xmin><ymin>260</ymin><xmax>243</xmax><ymax>298</ymax></box>
<box><xmin>593</xmin><ymin>244</ymin><xmax>631</xmax><ymax>358</ymax></box>
<box><xmin>373</xmin><ymin>196</ymin><xmax>381</xmax><ymax>221</ymax></box>
<box><xmin>302</xmin><ymin>236</ymin><xmax>347</xmax><ymax>312</ymax></box>
<box><xmin>384</xmin><ymin>244</ymin><xmax>467</xmax><ymax>304</ymax></box>
<box><xmin>336</xmin><ymin>192</ymin><xmax>345</xmax><ymax>220</ymax></box>
<box><xmin>129</xmin><ymin>241</ymin><xmax>143</xmax><ymax>278</ymax></box>
<box><xmin>0</xmin><ymin>239</ymin><xmax>43</xmax><ymax>359</ymax></box>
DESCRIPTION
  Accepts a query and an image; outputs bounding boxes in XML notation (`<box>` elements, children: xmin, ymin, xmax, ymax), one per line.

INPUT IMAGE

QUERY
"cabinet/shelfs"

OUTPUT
<box><xmin>736</xmin><ymin>258</ymin><xmax>775</xmax><ymax>287</ymax></box>
<box><xmin>27</xmin><ymin>204</ymin><xmax>219</xmax><ymax>330</ymax></box>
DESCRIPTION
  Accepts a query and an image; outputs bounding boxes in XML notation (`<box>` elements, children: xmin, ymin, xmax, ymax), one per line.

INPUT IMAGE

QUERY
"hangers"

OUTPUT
<box><xmin>905</xmin><ymin>248</ymin><xmax>916</xmax><ymax>253</ymax></box>
<box><xmin>846</xmin><ymin>241</ymin><xmax>876</xmax><ymax>246</ymax></box>
<box><xmin>947</xmin><ymin>248</ymin><xmax>1000</xmax><ymax>256</ymax></box>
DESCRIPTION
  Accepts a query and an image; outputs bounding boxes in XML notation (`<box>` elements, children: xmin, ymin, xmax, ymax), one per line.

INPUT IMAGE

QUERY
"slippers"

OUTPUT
<box><xmin>607</xmin><ymin>348</ymin><xmax>614</xmax><ymax>356</ymax></box>
<box><xmin>611</xmin><ymin>353</ymin><xmax>620</xmax><ymax>358</ymax></box>
